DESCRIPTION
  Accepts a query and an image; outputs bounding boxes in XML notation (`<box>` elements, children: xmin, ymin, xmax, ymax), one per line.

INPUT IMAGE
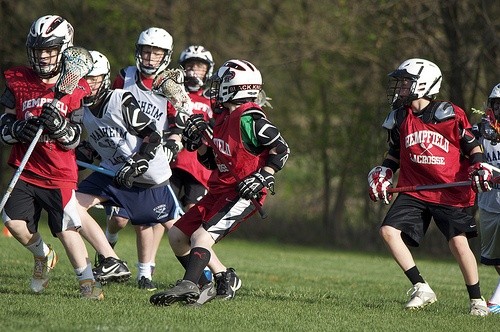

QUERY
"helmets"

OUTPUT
<box><xmin>134</xmin><ymin>27</ymin><xmax>174</xmax><ymax>75</ymax></box>
<box><xmin>178</xmin><ymin>45</ymin><xmax>214</xmax><ymax>91</ymax></box>
<box><xmin>80</xmin><ymin>50</ymin><xmax>111</xmax><ymax>106</ymax></box>
<box><xmin>210</xmin><ymin>59</ymin><xmax>263</xmax><ymax>114</ymax></box>
<box><xmin>386</xmin><ymin>57</ymin><xmax>442</xmax><ymax>109</ymax></box>
<box><xmin>487</xmin><ymin>83</ymin><xmax>500</xmax><ymax>124</ymax></box>
<box><xmin>25</xmin><ymin>15</ymin><xmax>74</xmax><ymax>79</ymax></box>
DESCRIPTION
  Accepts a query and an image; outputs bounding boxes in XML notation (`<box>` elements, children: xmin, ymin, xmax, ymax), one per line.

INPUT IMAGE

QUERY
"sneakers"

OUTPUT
<box><xmin>212</xmin><ymin>267</ymin><xmax>241</xmax><ymax>302</ymax></box>
<box><xmin>190</xmin><ymin>280</ymin><xmax>216</xmax><ymax>307</ymax></box>
<box><xmin>470</xmin><ymin>296</ymin><xmax>488</xmax><ymax>316</ymax></box>
<box><xmin>30</xmin><ymin>242</ymin><xmax>59</xmax><ymax>292</ymax></box>
<box><xmin>93</xmin><ymin>240</ymin><xmax>117</xmax><ymax>275</ymax></box>
<box><xmin>149</xmin><ymin>279</ymin><xmax>201</xmax><ymax>307</ymax></box>
<box><xmin>79</xmin><ymin>278</ymin><xmax>104</xmax><ymax>300</ymax></box>
<box><xmin>91</xmin><ymin>254</ymin><xmax>132</xmax><ymax>286</ymax></box>
<box><xmin>135</xmin><ymin>276</ymin><xmax>157</xmax><ymax>291</ymax></box>
<box><xmin>405</xmin><ymin>281</ymin><xmax>437</xmax><ymax>309</ymax></box>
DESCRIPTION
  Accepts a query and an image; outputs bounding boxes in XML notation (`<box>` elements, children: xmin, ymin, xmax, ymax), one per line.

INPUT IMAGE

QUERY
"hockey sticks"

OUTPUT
<box><xmin>256</xmin><ymin>87</ymin><xmax>267</xmax><ymax>108</ymax></box>
<box><xmin>385</xmin><ymin>175</ymin><xmax>500</xmax><ymax>193</ymax></box>
<box><xmin>152</xmin><ymin>68</ymin><xmax>268</xmax><ymax>220</ymax></box>
<box><xmin>75</xmin><ymin>160</ymin><xmax>116</xmax><ymax>176</ymax></box>
<box><xmin>0</xmin><ymin>46</ymin><xmax>95</xmax><ymax>214</ymax></box>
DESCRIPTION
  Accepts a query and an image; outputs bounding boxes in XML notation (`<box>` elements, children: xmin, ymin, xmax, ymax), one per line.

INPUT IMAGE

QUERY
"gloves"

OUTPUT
<box><xmin>114</xmin><ymin>152</ymin><xmax>150</xmax><ymax>189</ymax></box>
<box><xmin>181</xmin><ymin>113</ymin><xmax>216</xmax><ymax>152</ymax></box>
<box><xmin>75</xmin><ymin>140</ymin><xmax>95</xmax><ymax>171</ymax></box>
<box><xmin>12</xmin><ymin>118</ymin><xmax>51</xmax><ymax>143</ymax></box>
<box><xmin>467</xmin><ymin>163</ymin><xmax>493</xmax><ymax>193</ymax></box>
<box><xmin>368</xmin><ymin>166</ymin><xmax>393</xmax><ymax>205</ymax></box>
<box><xmin>39</xmin><ymin>102</ymin><xmax>72</xmax><ymax>139</ymax></box>
<box><xmin>235</xmin><ymin>167</ymin><xmax>275</xmax><ymax>200</ymax></box>
<box><xmin>162</xmin><ymin>138</ymin><xmax>182</xmax><ymax>164</ymax></box>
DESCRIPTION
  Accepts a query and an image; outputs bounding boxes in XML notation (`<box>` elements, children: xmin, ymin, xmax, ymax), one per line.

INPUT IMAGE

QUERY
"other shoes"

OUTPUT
<box><xmin>486</xmin><ymin>300</ymin><xmax>500</xmax><ymax>315</ymax></box>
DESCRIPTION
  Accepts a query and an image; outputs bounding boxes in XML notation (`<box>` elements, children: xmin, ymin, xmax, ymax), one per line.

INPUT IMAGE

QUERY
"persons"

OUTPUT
<box><xmin>0</xmin><ymin>16</ymin><xmax>291</xmax><ymax>306</ymax></box>
<box><xmin>368</xmin><ymin>58</ymin><xmax>500</xmax><ymax>315</ymax></box>
